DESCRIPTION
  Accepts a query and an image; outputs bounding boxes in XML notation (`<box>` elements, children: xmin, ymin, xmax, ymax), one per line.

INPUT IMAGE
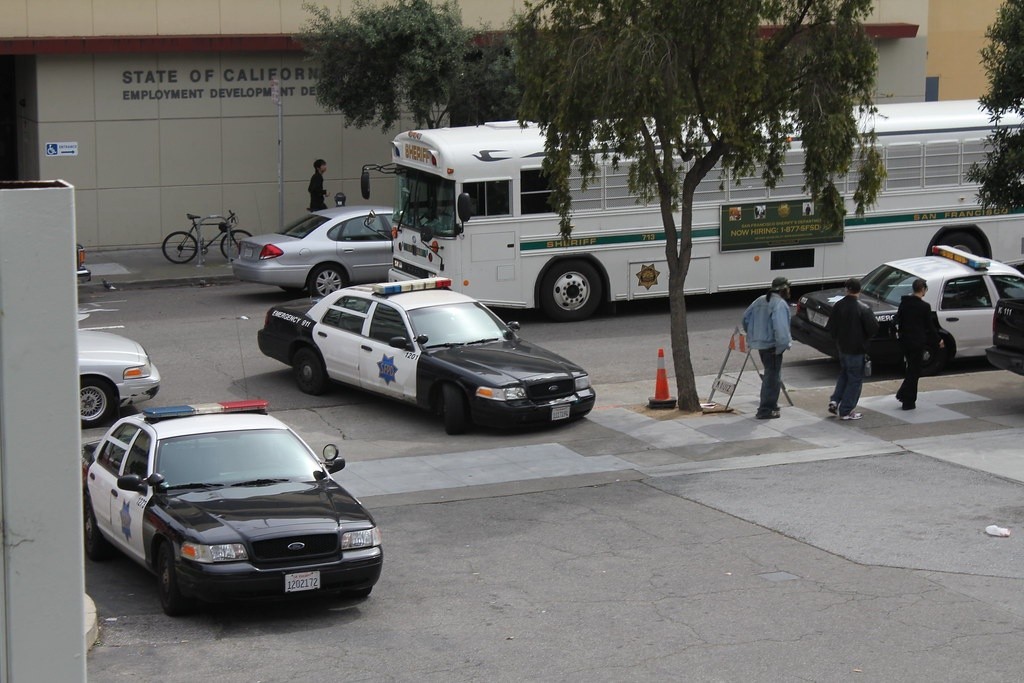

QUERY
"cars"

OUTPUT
<box><xmin>78</xmin><ymin>329</ymin><xmax>161</xmax><ymax>429</ymax></box>
<box><xmin>233</xmin><ymin>205</ymin><xmax>398</xmax><ymax>297</ymax></box>
<box><xmin>788</xmin><ymin>245</ymin><xmax>1024</xmax><ymax>383</ymax></box>
<box><xmin>256</xmin><ymin>276</ymin><xmax>597</xmax><ymax>436</ymax></box>
<box><xmin>82</xmin><ymin>400</ymin><xmax>382</xmax><ymax>618</ymax></box>
<box><xmin>983</xmin><ymin>298</ymin><xmax>1024</xmax><ymax>376</ymax></box>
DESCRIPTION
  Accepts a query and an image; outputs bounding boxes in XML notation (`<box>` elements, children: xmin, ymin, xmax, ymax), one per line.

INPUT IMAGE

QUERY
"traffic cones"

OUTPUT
<box><xmin>647</xmin><ymin>347</ymin><xmax>677</xmax><ymax>409</ymax></box>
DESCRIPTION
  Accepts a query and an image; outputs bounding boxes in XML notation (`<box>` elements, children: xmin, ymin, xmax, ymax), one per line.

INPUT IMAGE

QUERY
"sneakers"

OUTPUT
<box><xmin>828</xmin><ymin>401</ymin><xmax>838</xmax><ymax>416</ymax></box>
<box><xmin>840</xmin><ymin>411</ymin><xmax>863</xmax><ymax>420</ymax></box>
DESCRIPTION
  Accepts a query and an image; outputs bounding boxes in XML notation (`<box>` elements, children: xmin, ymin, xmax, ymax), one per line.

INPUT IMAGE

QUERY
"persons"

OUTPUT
<box><xmin>741</xmin><ymin>277</ymin><xmax>793</xmax><ymax>419</ymax></box>
<box><xmin>890</xmin><ymin>278</ymin><xmax>945</xmax><ymax>411</ymax></box>
<box><xmin>307</xmin><ymin>159</ymin><xmax>331</xmax><ymax>211</ymax></box>
<box><xmin>805</xmin><ymin>204</ymin><xmax>811</xmax><ymax>216</ymax></box>
<box><xmin>828</xmin><ymin>278</ymin><xmax>879</xmax><ymax>421</ymax></box>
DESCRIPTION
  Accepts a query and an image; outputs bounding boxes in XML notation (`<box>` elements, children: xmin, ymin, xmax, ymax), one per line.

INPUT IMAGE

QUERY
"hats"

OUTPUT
<box><xmin>770</xmin><ymin>277</ymin><xmax>791</xmax><ymax>291</ymax></box>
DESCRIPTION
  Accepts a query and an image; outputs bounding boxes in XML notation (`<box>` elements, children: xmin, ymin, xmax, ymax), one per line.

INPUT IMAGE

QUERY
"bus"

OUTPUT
<box><xmin>360</xmin><ymin>100</ymin><xmax>1023</xmax><ymax>322</ymax></box>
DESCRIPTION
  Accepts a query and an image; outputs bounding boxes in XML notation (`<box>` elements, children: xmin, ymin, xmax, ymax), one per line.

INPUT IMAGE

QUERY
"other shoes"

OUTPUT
<box><xmin>902</xmin><ymin>404</ymin><xmax>916</xmax><ymax>410</ymax></box>
<box><xmin>758</xmin><ymin>411</ymin><xmax>780</xmax><ymax>418</ymax></box>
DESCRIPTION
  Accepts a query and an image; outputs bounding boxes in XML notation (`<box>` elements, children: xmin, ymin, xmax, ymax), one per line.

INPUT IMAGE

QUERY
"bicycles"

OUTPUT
<box><xmin>162</xmin><ymin>210</ymin><xmax>253</xmax><ymax>264</ymax></box>
